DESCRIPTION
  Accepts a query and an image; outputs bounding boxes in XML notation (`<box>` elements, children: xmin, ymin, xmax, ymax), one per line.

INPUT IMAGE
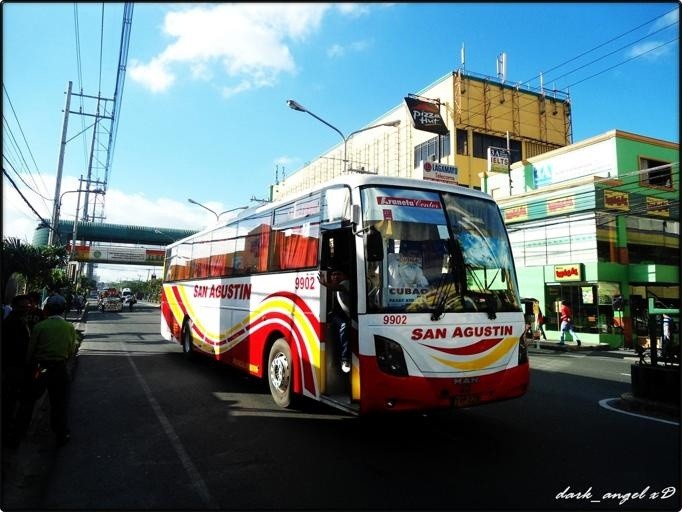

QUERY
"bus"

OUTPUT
<box><xmin>158</xmin><ymin>173</ymin><xmax>533</xmax><ymax>422</ymax></box>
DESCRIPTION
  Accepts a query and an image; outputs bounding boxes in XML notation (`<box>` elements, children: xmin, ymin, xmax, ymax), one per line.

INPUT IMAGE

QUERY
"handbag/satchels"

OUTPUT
<box><xmin>563</xmin><ymin>329</ymin><xmax>574</xmax><ymax>343</ymax></box>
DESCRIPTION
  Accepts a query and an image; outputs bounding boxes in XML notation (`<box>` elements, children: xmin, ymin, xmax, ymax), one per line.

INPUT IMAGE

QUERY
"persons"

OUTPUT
<box><xmin>376</xmin><ymin>239</ymin><xmax>428</xmax><ymax>304</ymax></box>
<box><xmin>0</xmin><ymin>289</ymin><xmax>89</xmax><ymax>328</ymax></box>
<box><xmin>316</xmin><ymin>267</ymin><xmax>371</xmax><ymax>373</ymax></box>
<box><xmin>28</xmin><ymin>295</ymin><xmax>76</xmax><ymax>445</ymax></box>
<box><xmin>558</xmin><ymin>300</ymin><xmax>581</xmax><ymax>346</ymax></box>
<box><xmin>0</xmin><ymin>296</ymin><xmax>37</xmax><ymax>451</ymax></box>
<box><xmin>537</xmin><ymin>307</ymin><xmax>547</xmax><ymax>340</ymax></box>
<box><xmin>125</xmin><ymin>294</ymin><xmax>133</xmax><ymax>311</ymax></box>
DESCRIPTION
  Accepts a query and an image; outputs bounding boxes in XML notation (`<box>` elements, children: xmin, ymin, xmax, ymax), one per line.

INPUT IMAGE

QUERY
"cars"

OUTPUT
<box><xmin>89</xmin><ymin>286</ymin><xmax>133</xmax><ymax>313</ymax></box>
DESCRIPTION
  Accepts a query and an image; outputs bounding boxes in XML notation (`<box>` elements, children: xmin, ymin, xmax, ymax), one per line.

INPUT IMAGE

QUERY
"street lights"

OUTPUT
<box><xmin>59</xmin><ymin>189</ymin><xmax>106</xmax><ymax>209</ymax></box>
<box><xmin>186</xmin><ymin>198</ymin><xmax>249</xmax><ymax>224</ymax></box>
<box><xmin>286</xmin><ymin>99</ymin><xmax>401</xmax><ymax>173</ymax></box>
<box><xmin>155</xmin><ymin>229</ymin><xmax>177</xmax><ymax>244</ymax></box>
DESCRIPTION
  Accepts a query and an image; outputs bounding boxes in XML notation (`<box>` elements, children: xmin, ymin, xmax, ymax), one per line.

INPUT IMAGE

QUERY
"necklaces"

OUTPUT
<box><xmin>662</xmin><ymin>312</ymin><xmax>675</xmax><ymax>366</ymax></box>
